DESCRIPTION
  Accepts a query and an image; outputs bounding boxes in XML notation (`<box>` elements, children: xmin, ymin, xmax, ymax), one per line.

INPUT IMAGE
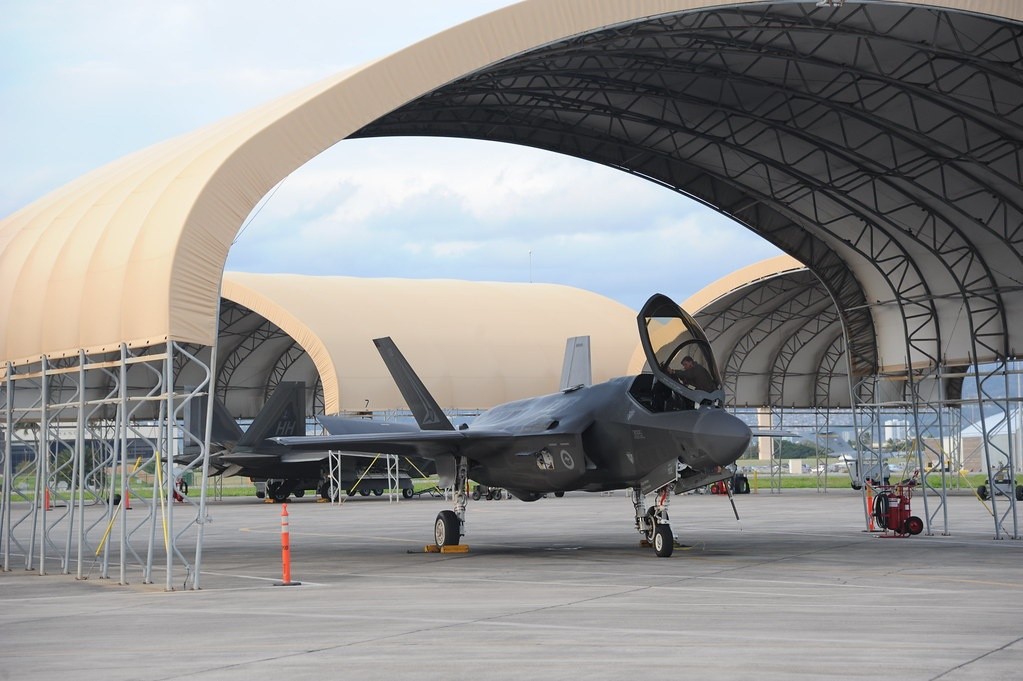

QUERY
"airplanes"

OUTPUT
<box><xmin>263</xmin><ymin>292</ymin><xmax>804</xmax><ymax>559</ymax></box>
<box><xmin>160</xmin><ymin>380</ymin><xmax>416</xmax><ymax>503</ymax></box>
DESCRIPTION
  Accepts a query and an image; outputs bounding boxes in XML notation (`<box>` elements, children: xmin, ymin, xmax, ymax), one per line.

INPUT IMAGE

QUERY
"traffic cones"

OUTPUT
<box><xmin>125</xmin><ymin>488</ymin><xmax>132</xmax><ymax>509</ymax></box>
<box><xmin>46</xmin><ymin>488</ymin><xmax>53</xmax><ymax>511</ymax></box>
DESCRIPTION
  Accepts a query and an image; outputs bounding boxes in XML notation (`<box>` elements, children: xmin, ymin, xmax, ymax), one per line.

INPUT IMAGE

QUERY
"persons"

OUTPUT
<box><xmin>666</xmin><ymin>356</ymin><xmax>715</xmax><ymax>409</ymax></box>
